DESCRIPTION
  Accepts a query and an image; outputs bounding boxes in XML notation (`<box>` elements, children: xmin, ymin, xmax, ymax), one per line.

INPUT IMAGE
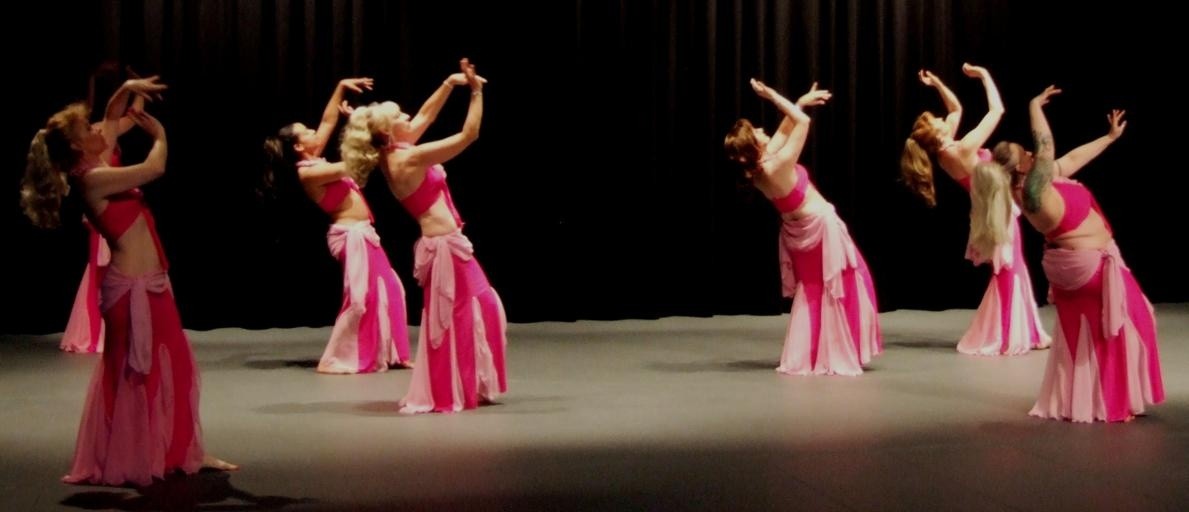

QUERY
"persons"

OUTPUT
<box><xmin>895</xmin><ymin>63</ymin><xmax>1055</xmax><ymax>358</ymax></box>
<box><xmin>340</xmin><ymin>58</ymin><xmax>510</xmax><ymax>415</ymax></box>
<box><xmin>254</xmin><ymin>76</ymin><xmax>412</xmax><ymax>373</ymax></box>
<box><xmin>18</xmin><ymin>74</ymin><xmax>239</xmax><ymax>485</ymax></box>
<box><xmin>723</xmin><ymin>76</ymin><xmax>884</xmax><ymax>374</ymax></box>
<box><xmin>968</xmin><ymin>82</ymin><xmax>1165</xmax><ymax>425</ymax></box>
<box><xmin>57</xmin><ymin>60</ymin><xmax>146</xmax><ymax>353</ymax></box>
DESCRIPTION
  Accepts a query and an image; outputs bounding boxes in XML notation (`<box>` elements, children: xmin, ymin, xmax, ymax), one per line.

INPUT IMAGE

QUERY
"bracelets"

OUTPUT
<box><xmin>442</xmin><ymin>80</ymin><xmax>455</xmax><ymax>90</ymax></box>
<box><xmin>470</xmin><ymin>90</ymin><xmax>483</xmax><ymax>96</ymax></box>
